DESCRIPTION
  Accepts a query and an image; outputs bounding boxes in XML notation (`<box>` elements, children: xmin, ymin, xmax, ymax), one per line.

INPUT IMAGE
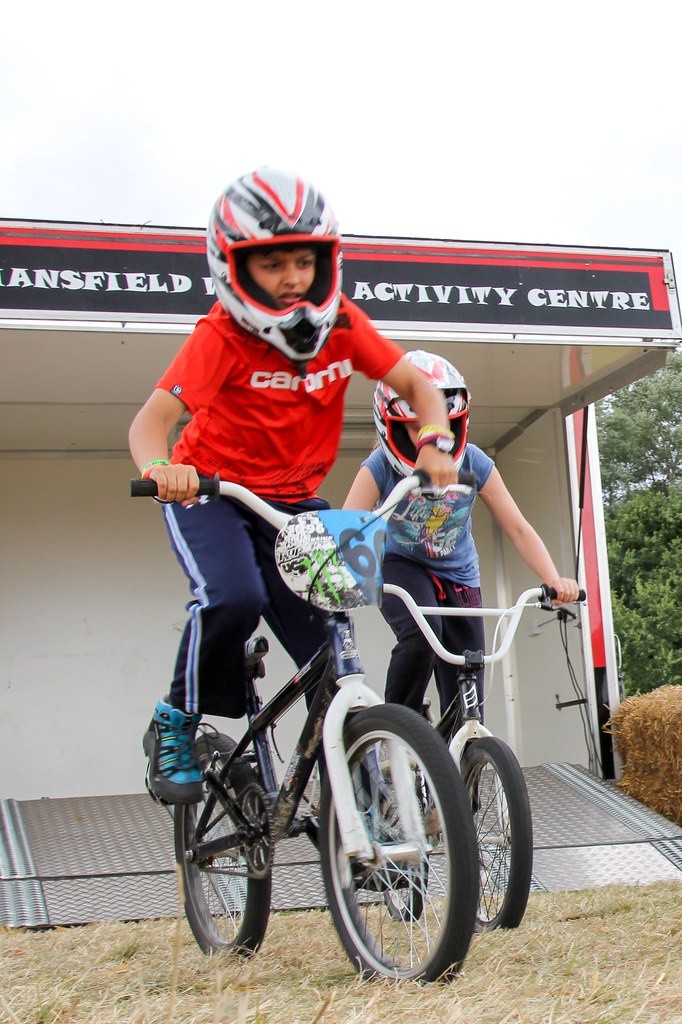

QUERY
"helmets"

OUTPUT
<box><xmin>373</xmin><ymin>350</ymin><xmax>470</xmax><ymax>478</ymax></box>
<box><xmin>206</xmin><ymin>168</ymin><xmax>343</xmax><ymax>362</ymax></box>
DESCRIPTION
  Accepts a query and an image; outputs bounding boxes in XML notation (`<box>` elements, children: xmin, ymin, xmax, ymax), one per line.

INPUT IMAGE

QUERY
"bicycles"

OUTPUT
<box><xmin>382</xmin><ymin>581</ymin><xmax>586</xmax><ymax>935</ymax></box>
<box><xmin>127</xmin><ymin>469</ymin><xmax>480</xmax><ymax>987</ymax></box>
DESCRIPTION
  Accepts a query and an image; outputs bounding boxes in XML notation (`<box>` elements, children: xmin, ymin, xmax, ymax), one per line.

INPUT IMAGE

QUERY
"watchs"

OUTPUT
<box><xmin>427</xmin><ymin>437</ymin><xmax>455</xmax><ymax>456</ymax></box>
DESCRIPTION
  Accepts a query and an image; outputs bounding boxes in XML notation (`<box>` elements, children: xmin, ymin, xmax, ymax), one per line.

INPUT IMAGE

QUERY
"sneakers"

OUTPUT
<box><xmin>143</xmin><ymin>693</ymin><xmax>205</xmax><ymax>804</ymax></box>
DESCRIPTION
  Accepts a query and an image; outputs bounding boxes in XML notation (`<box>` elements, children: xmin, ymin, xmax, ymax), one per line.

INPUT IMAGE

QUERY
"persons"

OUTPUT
<box><xmin>129</xmin><ymin>168</ymin><xmax>460</xmax><ymax>801</ymax></box>
<box><xmin>342</xmin><ymin>350</ymin><xmax>579</xmax><ymax>807</ymax></box>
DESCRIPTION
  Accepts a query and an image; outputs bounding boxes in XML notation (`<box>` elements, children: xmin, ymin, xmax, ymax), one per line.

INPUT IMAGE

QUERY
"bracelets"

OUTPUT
<box><xmin>415</xmin><ymin>427</ymin><xmax>456</xmax><ymax>452</ymax></box>
<box><xmin>141</xmin><ymin>459</ymin><xmax>174</xmax><ymax>480</ymax></box>
